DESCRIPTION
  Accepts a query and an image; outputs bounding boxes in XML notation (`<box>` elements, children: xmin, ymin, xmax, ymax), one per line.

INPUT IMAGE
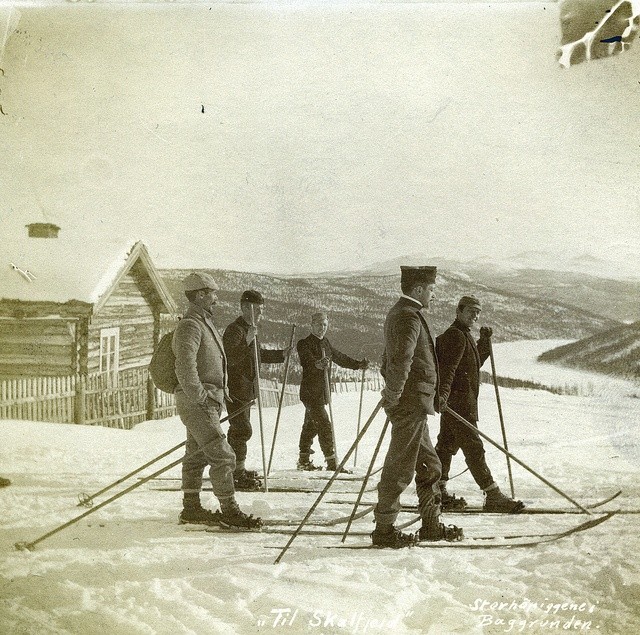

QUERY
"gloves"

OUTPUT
<box><xmin>479</xmin><ymin>327</ymin><xmax>492</xmax><ymax>344</ymax></box>
<box><xmin>439</xmin><ymin>397</ymin><xmax>446</xmax><ymax>411</ymax></box>
<box><xmin>381</xmin><ymin>386</ymin><xmax>402</xmax><ymax>409</ymax></box>
<box><xmin>358</xmin><ymin>360</ymin><xmax>370</xmax><ymax>370</ymax></box>
<box><xmin>315</xmin><ymin>357</ymin><xmax>330</xmax><ymax>370</ymax></box>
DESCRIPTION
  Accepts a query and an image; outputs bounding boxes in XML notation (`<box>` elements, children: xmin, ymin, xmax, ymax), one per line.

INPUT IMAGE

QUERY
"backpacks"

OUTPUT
<box><xmin>148</xmin><ymin>329</ymin><xmax>178</xmax><ymax>394</ymax></box>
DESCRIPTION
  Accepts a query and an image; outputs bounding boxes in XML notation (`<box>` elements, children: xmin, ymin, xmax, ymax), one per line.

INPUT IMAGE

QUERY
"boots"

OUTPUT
<box><xmin>234</xmin><ymin>461</ymin><xmax>262</xmax><ymax>489</ymax></box>
<box><xmin>483</xmin><ymin>486</ymin><xmax>526</xmax><ymax>512</ymax></box>
<box><xmin>246</xmin><ymin>471</ymin><xmax>258</xmax><ymax>478</ymax></box>
<box><xmin>180</xmin><ymin>499</ymin><xmax>222</xmax><ymax>525</ymax></box>
<box><xmin>219</xmin><ymin>502</ymin><xmax>263</xmax><ymax>530</ymax></box>
<box><xmin>415</xmin><ymin>504</ymin><xmax>464</xmax><ymax>541</ymax></box>
<box><xmin>298</xmin><ymin>452</ymin><xmax>322</xmax><ymax>471</ymax></box>
<box><xmin>324</xmin><ymin>452</ymin><xmax>348</xmax><ymax>473</ymax></box>
<box><xmin>370</xmin><ymin>510</ymin><xmax>416</xmax><ymax>549</ymax></box>
<box><xmin>440</xmin><ymin>485</ymin><xmax>467</xmax><ymax>511</ymax></box>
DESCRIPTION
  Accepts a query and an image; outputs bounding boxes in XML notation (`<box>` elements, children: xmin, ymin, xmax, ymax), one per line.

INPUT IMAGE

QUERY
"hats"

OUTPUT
<box><xmin>458</xmin><ymin>295</ymin><xmax>481</xmax><ymax>311</ymax></box>
<box><xmin>182</xmin><ymin>273</ymin><xmax>220</xmax><ymax>291</ymax></box>
<box><xmin>241</xmin><ymin>291</ymin><xmax>264</xmax><ymax>304</ymax></box>
<box><xmin>312</xmin><ymin>313</ymin><xmax>327</xmax><ymax>324</ymax></box>
<box><xmin>400</xmin><ymin>266</ymin><xmax>437</xmax><ymax>282</ymax></box>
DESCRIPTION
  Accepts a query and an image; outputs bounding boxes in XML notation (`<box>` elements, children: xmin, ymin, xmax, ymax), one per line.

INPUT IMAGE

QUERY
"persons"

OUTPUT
<box><xmin>434</xmin><ymin>293</ymin><xmax>525</xmax><ymax>513</ymax></box>
<box><xmin>297</xmin><ymin>313</ymin><xmax>370</xmax><ymax>473</ymax></box>
<box><xmin>221</xmin><ymin>291</ymin><xmax>294</xmax><ymax>491</ymax></box>
<box><xmin>369</xmin><ymin>267</ymin><xmax>463</xmax><ymax>548</ymax></box>
<box><xmin>173</xmin><ymin>272</ymin><xmax>264</xmax><ymax>529</ymax></box>
